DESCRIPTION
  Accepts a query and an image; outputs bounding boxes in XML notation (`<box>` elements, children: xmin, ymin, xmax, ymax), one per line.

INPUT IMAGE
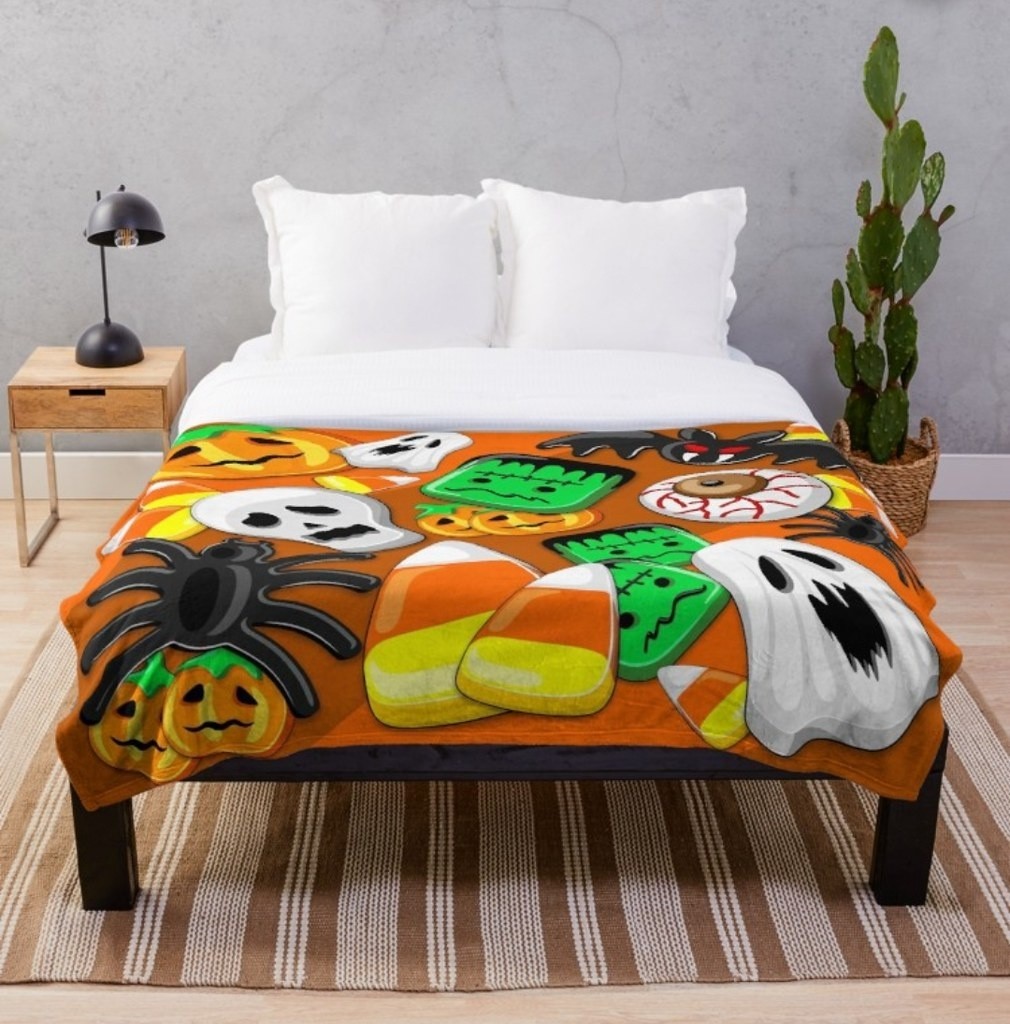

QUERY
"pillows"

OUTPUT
<box><xmin>252</xmin><ymin>176</ymin><xmax>499</xmax><ymax>346</ymax></box>
<box><xmin>482</xmin><ymin>178</ymin><xmax>749</xmax><ymax>359</ymax></box>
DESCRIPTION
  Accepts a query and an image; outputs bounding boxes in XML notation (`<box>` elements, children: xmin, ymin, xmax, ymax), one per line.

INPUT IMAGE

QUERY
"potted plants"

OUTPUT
<box><xmin>827</xmin><ymin>26</ymin><xmax>955</xmax><ymax>535</ymax></box>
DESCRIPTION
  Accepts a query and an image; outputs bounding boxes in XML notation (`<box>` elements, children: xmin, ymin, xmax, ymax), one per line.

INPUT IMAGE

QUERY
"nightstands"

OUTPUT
<box><xmin>6</xmin><ymin>345</ymin><xmax>187</xmax><ymax>567</ymax></box>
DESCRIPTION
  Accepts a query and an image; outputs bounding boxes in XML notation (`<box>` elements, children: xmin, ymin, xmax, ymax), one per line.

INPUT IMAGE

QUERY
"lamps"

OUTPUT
<box><xmin>75</xmin><ymin>184</ymin><xmax>166</xmax><ymax>369</ymax></box>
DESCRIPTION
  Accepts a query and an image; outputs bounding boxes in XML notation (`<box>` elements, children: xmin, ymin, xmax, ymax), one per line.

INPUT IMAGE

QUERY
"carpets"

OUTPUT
<box><xmin>1</xmin><ymin>666</ymin><xmax>1009</xmax><ymax>993</ymax></box>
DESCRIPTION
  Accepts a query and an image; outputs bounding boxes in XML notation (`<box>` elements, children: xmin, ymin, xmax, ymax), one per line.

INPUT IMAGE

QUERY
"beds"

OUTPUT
<box><xmin>57</xmin><ymin>327</ymin><xmax>963</xmax><ymax>909</ymax></box>
